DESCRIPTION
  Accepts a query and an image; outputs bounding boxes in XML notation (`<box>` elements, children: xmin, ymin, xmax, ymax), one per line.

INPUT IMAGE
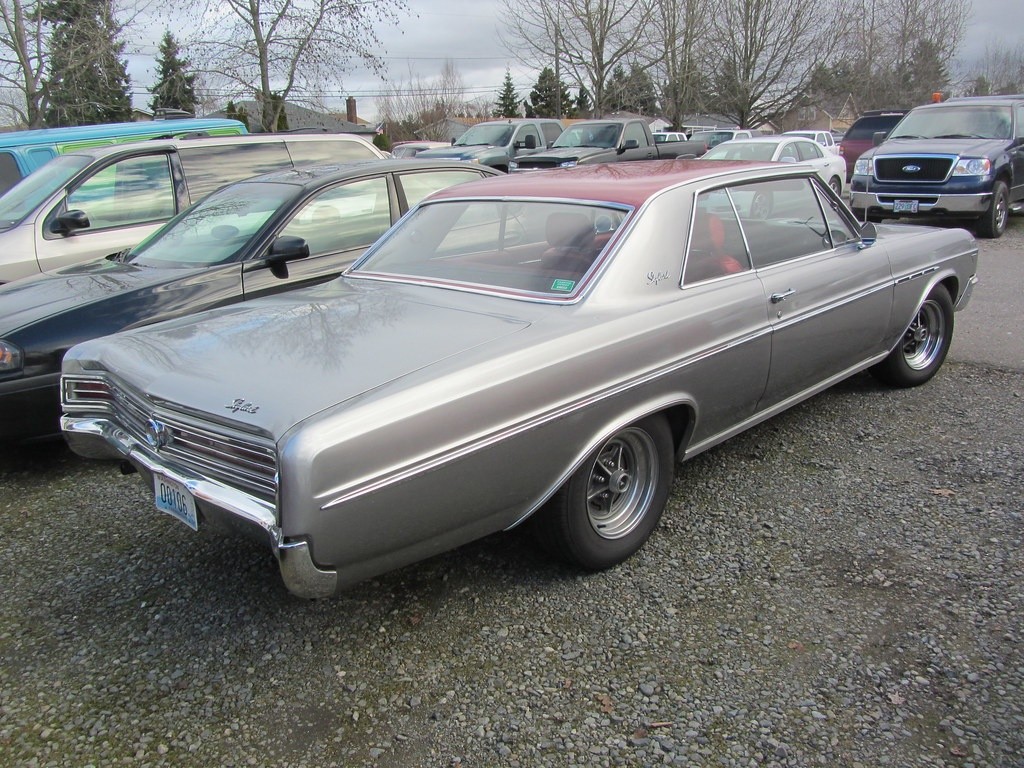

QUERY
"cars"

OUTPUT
<box><xmin>383</xmin><ymin>142</ymin><xmax>454</xmax><ymax>159</ymax></box>
<box><xmin>58</xmin><ymin>153</ymin><xmax>977</xmax><ymax>599</ymax></box>
<box><xmin>695</xmin><ymin>135</ymin><xmax>848</xmax><ymax>219</ymax></box>
<box><xmin>0</xmin><ymin>160</ymin><xmax>526</xmax><ymax>440</ymax></box>
<box><xmin>568</xmin><ymin>129</ymin><xmax>593</xmax><ymax>145</ymax></box>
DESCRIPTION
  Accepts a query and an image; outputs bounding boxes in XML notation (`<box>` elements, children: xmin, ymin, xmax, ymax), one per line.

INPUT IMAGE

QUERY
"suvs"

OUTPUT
<box><xmin>838</xmin><ymin>109</ymin><xmax>908</xmax><ymax>183</ymax></box>
<box><xmin>850</xmin><ymin>95</ymin><xmax>1024</xmax><ymax>238</ymax></box>
<box><xmin>688</xmin><ymin>129</ymin><xmax>766</xmax><ymax>160</ymax></box>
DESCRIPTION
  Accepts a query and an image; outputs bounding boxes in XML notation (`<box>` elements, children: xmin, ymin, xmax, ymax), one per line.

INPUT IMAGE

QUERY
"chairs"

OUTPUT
<box><xmin>538</xmin><ymin>212</ymin><xmax>596</xmax><ymax>294</ymax></box>
<box><xmin>587</xmin><ymin>232</ymin><xmax>614</xmax><ymax>249</ymax></box>
<box><xmin>689</xmin><ymin>209</ymin><xmax>744</xmax><ymax>278</ymax></box>
<box><xmin>740</xmin><ymin>148</ymin><xmax>753</xmax><ymax>160</ymax></box>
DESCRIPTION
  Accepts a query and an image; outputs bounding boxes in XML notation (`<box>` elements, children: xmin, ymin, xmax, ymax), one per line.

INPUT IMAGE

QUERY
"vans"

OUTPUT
<box><xmin>0</xmin><ymin>116</ymin><xmax>255</xmax><ymax>207</ymax></box>
<box><xmin>0</xmin><ymin>132</ymin><xmax>392</xmax><ymax>283</ymax></box>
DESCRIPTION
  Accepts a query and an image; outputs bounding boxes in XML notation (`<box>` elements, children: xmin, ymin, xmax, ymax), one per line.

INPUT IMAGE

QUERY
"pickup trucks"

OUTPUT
<box><xmin>651</xmin><ymin>133</ymin><xmax>688</xmax><ymax>142</ymax></box>
<box><xmin>417</xmin><ymin>118</ymin><xmax>566</xmax><ymax>175</ymax></box>
<box><xmin>780</xmin><ymin>130</ymin><xmax>841</xmax><ymax>156</ymax></box>
<box><xmin>506</xmin><ymin>114</ymin><xmax>708</xmax><ymax>175</ymax></box>
